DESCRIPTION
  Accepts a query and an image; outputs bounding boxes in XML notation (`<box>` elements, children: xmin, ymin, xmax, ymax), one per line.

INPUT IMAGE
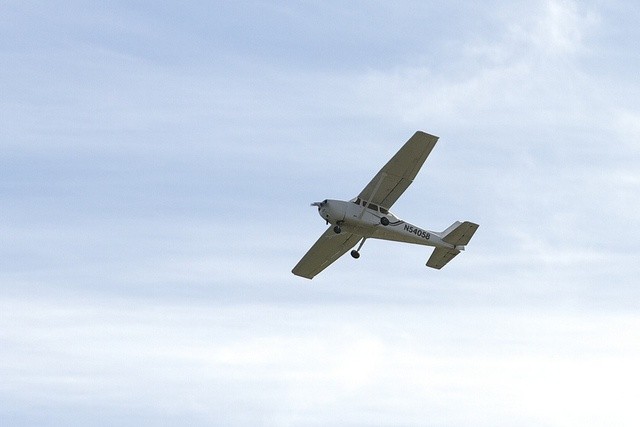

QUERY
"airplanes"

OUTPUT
<box><xmin>292</xmin><ymin>131</ymin><xmax>479</xmax><ymax>279</ymax></box>
